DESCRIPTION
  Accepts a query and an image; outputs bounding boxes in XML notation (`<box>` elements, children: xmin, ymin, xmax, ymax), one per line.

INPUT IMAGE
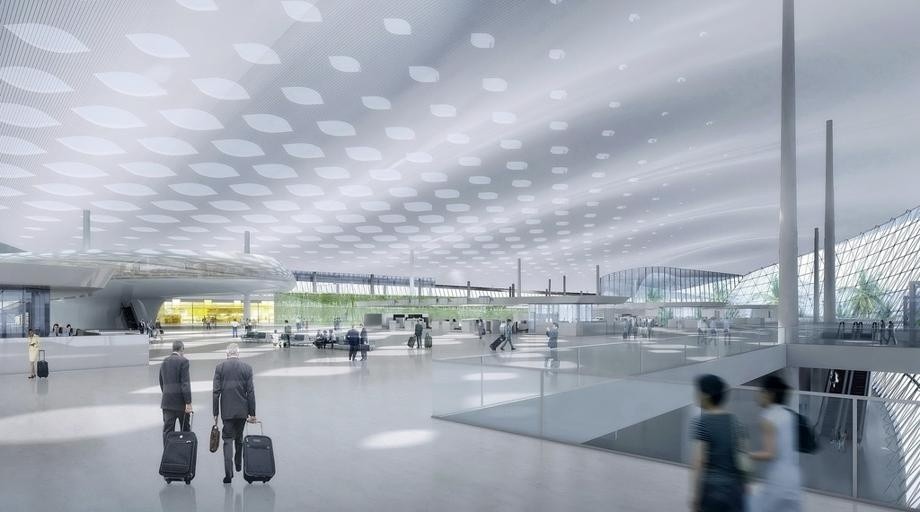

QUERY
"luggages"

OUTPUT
<box><xmin>243</xmin><ymin>419</ymin><xmax>276</xmax><ymax>484</ymax></box>
<box><xmin>159</xmin><ymin>410</ymin><xmax>197</xmax><ymax>484</ymax></box>
<box><xmin>408</xmin><ymin>335</ymin><xmax>417</xmax><ymax>348</ymax></box>
<box><xmin>425</xmin><ymin>333</ymin><xmax>432</xmax><ymax>348</ymax></box>
<box><xmin>37</xmin><ymin>350</ymin><xmax>48</xmax><ymax>378</ymax></box>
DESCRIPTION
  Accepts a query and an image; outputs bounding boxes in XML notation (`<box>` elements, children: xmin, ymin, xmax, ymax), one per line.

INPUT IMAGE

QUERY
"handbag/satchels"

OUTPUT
<box><xmin>358</xmin><ymin>343</ymin><xmax>370</xmax><ymax>351</ymax></box>
<box><xmin>210</xmin><ymin>425</ymin><xmax>220</xmax><ymax>452</ymax></box>
<box><xmin>784</xmin><ymin>407</ymin><xmax>818</xmax><ymax>454</ymax></box>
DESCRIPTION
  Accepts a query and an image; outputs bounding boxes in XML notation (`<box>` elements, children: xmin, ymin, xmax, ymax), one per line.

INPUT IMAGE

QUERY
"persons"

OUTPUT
<box><xmin>360</xmin><ymin>323</ymin><xmax>370</xmax><ymax>361</ymax></box>
<box><xmin>284</xmin><ymin>320</ymin><xmax>292</xmax><ymax>349</ymax></box>
<box><xmin>26</xmin><ymin>328</ymin><xmax>40</xmax><ymax>379</ymax></box>
<box><xmin>313</xmin><ymin>329</ymin><xmax>323</xmax><ymax>349</ymax></box>
<box><xmin>697</xmin><ymin>317</ymin><xmax>703</xmax><ymax>344</ymax></box>
<box><xmin>212</xmin><ymin>343</ymin><xmax>256</xmax><ymax>484</ymax></box>
<box><xmin>546</xmin><ymin>323</ymin><xmax>558</xmax><ymax>346</ymax></box>
<box><xmin>322</xmin><ymin>330</ymin><xmax>328</xmax><ymax>348</ymax></box>
<box><xmin>48</xmin><ymin>324</ymin><xmax>63</xmax><ymax>336</ymax></box>
<box><xmin>622</xmin><ymin>316</ymin><xmax>654</xmax><ymax>339</ymax></box>
<box><xmin>327</xmin><ymin>329</ymin><xmax>336</xmax><ymax>350</ymax></box>
<box><xmin>879</xmin><ymin>318</ymin><xmax>888</xmax><ymax>344</ymax></box>
<box><xmin>500</xmin><ymin>318</ymin><xmax>515</xmax><ymax>350</ymax></box>
<box><xmin>66</xmin><ymin>323</ymin><xmax>71</xmax><ymax>328</ymax></box>
<box><xmin>745</xmin><ymin>375</ymin><xmax>805</xmax><ymax>511</ymax></box>
<box><xmin>346</xmin><ymin>324</ymin><xmax>360</xmax><ymax>362</ymax></box>
<box><xmin>245</xmin><ymin>316</ymin><xmax>258</xmax><ymax>333</ymax></box>
<box><xmin>702</xmin><ymin>319</ymin><xmax>708</xmax><ymax>345</ymax></box>
<box><xmin>709</xmin><ymin>317</ymin><xmax>718</xmax><ymax>345</ymax></box>
<box><xmin>885</xmin><ymin>321</ymin><xmax>897</xmax><ymax>344</ymax></box>
<box><xmin>159</xmin><ymin>340</ymin><xmax>192</xmax><ymax>485</ymax></box>
<box><xmin>685</xmin><ymin>376</ymin><xmax>748</xmax><ymax>511</ymax></box>
<box><xmin>271</xmin><ymin>330</ymin><xmax>282</xmax><ymax>351</ymax></box>
<box><xmin>147</xmin><ymin>320</ymin><xmax>156</xmax><ymax>337</ymax></box>
<box><xmin>415</xmin><ymin>319</ymin><xmax>423</xmax><ymax>347</ymax></box>
<box><xmin>232</xmin><ymin>319</ymin><xmax>240</xmax><ymax>338</ymax></box>
<box><xmin>722</xmin><ymin>317</ymin><xmax>732</xmax><ymax>344</ymax></box>
<box><xmin>223</xmin><ymin>483</ymin><xmax>243</xmax><ymax>511</ymax></box>
<box><xmin>206</xmin><ymin>316</ymin><xmax>211</xmax><ymax>329</ymax></box>
<box><xmin>475</xmin><ymin>314</ymin><xmax>485</xmax><ymax>340</ymax></box>
<box><xmin>139</xmin><ymin>319</ymin><xmax>146</xmax><ymax>333</ymax></box>
<box><xmin>202</xmin><ymin>316</ymin><xmax>206</xmax><ymax>329</ymax></box>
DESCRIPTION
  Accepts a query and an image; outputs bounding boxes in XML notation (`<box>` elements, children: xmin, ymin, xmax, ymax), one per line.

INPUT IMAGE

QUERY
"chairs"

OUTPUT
<box><xmin>43</xmin><ymin>321</ymin><xmax>102</xmax><ymax>339</ymax></box>
<box><xmin>233</xmin><ymin>329</ymin><xmax>373</xmax><ymax>351</ymax></box>
